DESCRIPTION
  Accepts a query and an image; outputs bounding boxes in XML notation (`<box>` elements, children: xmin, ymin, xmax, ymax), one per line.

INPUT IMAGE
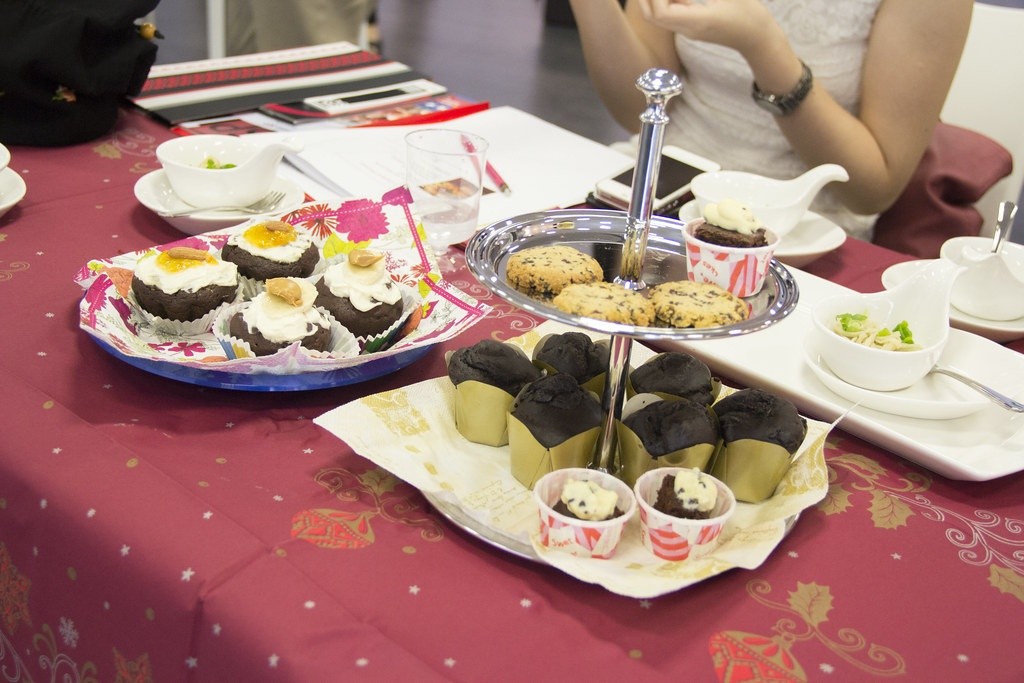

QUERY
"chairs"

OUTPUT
<box><xmin>939</xmin><ymin>2</ymin><xmax>1024</xmax><ymax>239</ymax></box>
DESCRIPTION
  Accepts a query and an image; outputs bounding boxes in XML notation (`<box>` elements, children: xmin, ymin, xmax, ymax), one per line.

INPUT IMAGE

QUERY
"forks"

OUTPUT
<box><xmin>158</xmin><ymin>190</ymin><xmax>286</xmax><ymax>219</ymax></box>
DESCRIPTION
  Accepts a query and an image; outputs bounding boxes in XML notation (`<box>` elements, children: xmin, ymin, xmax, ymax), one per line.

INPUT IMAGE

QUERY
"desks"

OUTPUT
<box><xmin>0</xmin><ymin>102</ymin><xmax>1024</xmax><ymax>683</ymax></box>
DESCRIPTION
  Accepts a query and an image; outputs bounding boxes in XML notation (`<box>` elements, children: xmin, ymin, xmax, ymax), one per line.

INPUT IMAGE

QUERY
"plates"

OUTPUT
<box><xmin>680</xmin><ymin>198</ymin><xmax>847</xmax><ymax>269</ymax></box>
<box><xmin>84</xmin><ymin>290</ymin><xmax>437</xmax><ymax>392</ymax></box>
<box><xmin>0</xmin><ymin>166</ymin><xmax>26</xmax><ymax>219</ymax></box>
<box><xmin>805</xmin><ymin>339</ymin><xmax>1001</xmax><ymax>419</ymax></box>
<box><xmin>645</xmin><ymin>263</ymin><xmax>1024</xmax><ymax>484</ymax></box>
<box><xmin>881</xmin><ymin>258</ymin><xmax>1024</xmax><ymax>344</ymax></box>
<box><xmin>134</xmin><ymin>169</ymin><xmax>305</xmax><ymax>236</ymax></box>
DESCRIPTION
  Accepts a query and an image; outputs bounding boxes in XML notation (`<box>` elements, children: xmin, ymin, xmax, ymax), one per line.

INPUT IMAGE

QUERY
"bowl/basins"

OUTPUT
<box><xmin>0</xmin><ymin>142</ymin><xmax>12</xmax><ymax>171</ymax></box>
<box><xmin>940</xmin><ymin>235</ymin><xmax>1023</xmax><ymax>321</ymax></box>
<box><xmin>817</xmin><ymin>254</ymin><xmax>969</xmax><ymax>392</ymax></box>
<box><xmin>157</xmin><ymin>135</ymin><xmax>285</xmax><ymax>211</ymax></box>
<box><xmin>691</xmin><ymin>163</ymin><xmax>850</xmax><ymax>239</ymax></box>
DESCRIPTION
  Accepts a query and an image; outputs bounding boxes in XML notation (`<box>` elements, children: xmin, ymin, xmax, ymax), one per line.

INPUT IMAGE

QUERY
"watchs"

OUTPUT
<box><xmin>752</xmin><ymin>59</ymin><xmax>813</xmax><ymax>114</ymax></box>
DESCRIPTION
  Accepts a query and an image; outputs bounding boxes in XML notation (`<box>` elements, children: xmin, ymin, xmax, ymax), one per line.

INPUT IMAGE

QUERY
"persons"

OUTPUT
<box><xmin>568</xmin><ymin>0</ymin><xmax>974</xmax><ymax>240</ymax></box>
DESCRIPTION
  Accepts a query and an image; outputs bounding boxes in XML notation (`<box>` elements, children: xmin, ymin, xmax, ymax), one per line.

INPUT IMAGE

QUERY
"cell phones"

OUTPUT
<box><xmin>597</xmin><ymin>145</ymin><xmax>721</xmax><ymax>213</ymax></box>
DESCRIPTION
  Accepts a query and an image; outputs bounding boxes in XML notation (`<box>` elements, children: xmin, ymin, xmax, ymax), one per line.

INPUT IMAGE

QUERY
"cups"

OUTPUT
<box><xmin>404</xmin><ymin>128</ymin><xmax>489</xmax><ymax>246</ymax></box>
<box><xmin>533</xmin><ymin>468</ymin><xmax>636</xmax><ymax>561</ymax></box>
<box><xmin>682</xmin><ymin>218</ymin><xmax>781</xmax><ymax>298</ymax></box>
<box><xmin>633</xmin><ymin>466</ymin><xmax>737</xmax><ymax>562</ymax></box>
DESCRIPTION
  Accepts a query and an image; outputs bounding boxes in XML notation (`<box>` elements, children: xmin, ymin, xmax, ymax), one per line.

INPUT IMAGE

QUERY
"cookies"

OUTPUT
<box><xmin>509</xmin><ymin>244</ymin><xmax>750</xmax><ymax>330</ymax></box>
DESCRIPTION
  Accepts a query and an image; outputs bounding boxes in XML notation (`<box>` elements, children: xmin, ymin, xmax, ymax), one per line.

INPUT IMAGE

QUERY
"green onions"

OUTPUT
<box><xmin>837</xmin><ymin>312</ymin><xmax>916</xmax><ymax>346</ymax></box>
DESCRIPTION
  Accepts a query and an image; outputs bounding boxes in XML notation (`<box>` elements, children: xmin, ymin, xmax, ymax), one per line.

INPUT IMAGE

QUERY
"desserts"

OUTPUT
<box><xmin>682</xmin><ymin>196</ymin><xmax>778</xmax><ymax>300</ymax></box>
<box><xmin>125</xmin><ymin>220</ymin><xmax>418</xmax><ymax>360</ymax></box>
<box><xmin>442</xmin><ymin>331</ymin><xmax>809</xmax><ymax>563</ymax></box>
